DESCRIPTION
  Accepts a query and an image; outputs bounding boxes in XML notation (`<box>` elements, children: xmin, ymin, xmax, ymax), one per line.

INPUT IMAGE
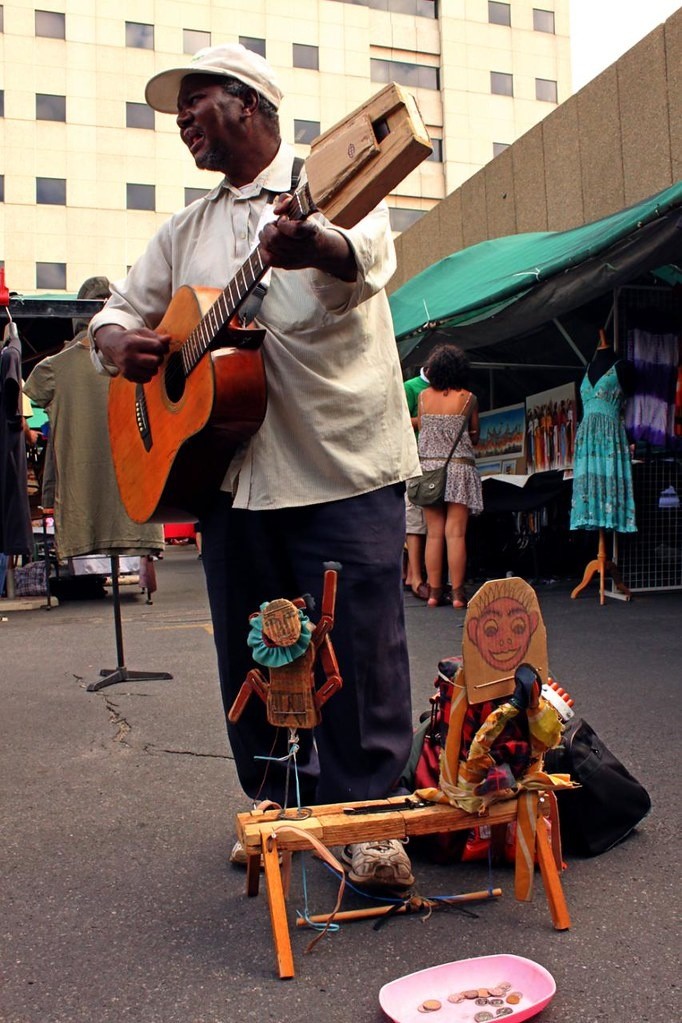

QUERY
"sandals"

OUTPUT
<box><xmin>427</xmin><ymin>586</ymin><xmax>452</xmax><ymax>606</ymax></box>
<box><xmin>451</xmin><ymin>584</ymin><xmax>475</xmax><ymax>611</ymax></box>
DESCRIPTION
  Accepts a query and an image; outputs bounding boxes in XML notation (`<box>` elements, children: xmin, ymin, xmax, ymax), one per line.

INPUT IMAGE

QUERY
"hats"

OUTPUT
<box><xmin>145</xmin><ymin>41</ymin><xmax>284</xmax><ymax>116</ymax></box>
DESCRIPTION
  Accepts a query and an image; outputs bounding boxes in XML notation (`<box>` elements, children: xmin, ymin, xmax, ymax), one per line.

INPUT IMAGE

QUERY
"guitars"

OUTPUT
<box><xmin>105</xmin><ymin>120</ymin><xmax>391</xmax><ymax>526</ymax></box>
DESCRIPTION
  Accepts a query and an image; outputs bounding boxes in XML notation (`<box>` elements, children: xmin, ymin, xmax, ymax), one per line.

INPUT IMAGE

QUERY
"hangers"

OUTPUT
<box><xmin>0</xmin><ymin>295</ymin><xmax>25</xmax><ymax>347</ymax></box>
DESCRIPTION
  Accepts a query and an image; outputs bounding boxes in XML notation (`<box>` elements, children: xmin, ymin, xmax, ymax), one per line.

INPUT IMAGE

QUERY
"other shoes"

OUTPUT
<box><xmin>412</xmin><ymin>582</ymin><xmax>431</xmax><ymax>601</ymax></box>
<box><xmin>403</xmin><ymin>580</ymin><xmax>413</xmax><ymax>591</ymax></box>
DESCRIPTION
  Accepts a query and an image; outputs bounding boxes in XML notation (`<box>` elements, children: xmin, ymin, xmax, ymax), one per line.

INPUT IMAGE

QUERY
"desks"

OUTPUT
<box><xmin>482</xmin><ymin>469</ymin><xmax>573</xmax><ymax>586</ymax></box>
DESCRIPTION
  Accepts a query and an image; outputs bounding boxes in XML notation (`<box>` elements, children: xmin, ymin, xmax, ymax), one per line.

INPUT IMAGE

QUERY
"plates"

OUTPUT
<box><xmin>378</xmin><ymin>954</ymin><xmax>556</xmax><ymax>1023</ymax></box>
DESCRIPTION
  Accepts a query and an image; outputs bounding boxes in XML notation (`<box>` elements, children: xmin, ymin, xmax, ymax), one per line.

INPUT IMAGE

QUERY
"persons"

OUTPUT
<box><xmin>402</xmin><ymin>364</ymin><xmax>430</xmax><ymax>599</ymax></box>
<box><xmin>417</xmin><ymin>345</ymin><xmax>483</xmax><ymax>609</ymax></box>
<box><xmin>85</xmin><ymin>43</ymin><xmax>424</xmax><ymax>885</ymax></box>
<box><xmin>569</xmin><ymin>346</ymin><xmax>637</xmax><ymax>533</ymax></box>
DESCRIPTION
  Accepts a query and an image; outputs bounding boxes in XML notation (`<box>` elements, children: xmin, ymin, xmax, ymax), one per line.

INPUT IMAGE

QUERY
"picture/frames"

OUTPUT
<box><xmin>502</xmin><ymin>459</ymin><xmax>516</xmax><ymax>474</ymax></box>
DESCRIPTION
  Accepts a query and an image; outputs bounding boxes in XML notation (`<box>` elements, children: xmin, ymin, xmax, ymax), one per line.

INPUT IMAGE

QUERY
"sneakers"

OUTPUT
<box><xmin>342</xmin><ymin>835</ymin><xmax>418</xmax><ymax>885</ymax></box>
<box><xmin>230</xmin><ymin>830</ymin><xmax>285</xmax><ymax>868</ymax></box>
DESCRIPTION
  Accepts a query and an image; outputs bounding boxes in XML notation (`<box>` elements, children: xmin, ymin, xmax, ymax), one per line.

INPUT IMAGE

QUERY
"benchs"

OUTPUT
<box><xmin>238</xmin><ymin>794</ymin><xmax>571</xmax><ymax>982</ymax></box>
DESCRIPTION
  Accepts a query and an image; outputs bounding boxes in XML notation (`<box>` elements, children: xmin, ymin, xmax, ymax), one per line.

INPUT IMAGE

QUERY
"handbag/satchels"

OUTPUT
<box><xmin>406</xmin><ymin>466</ymin><xmax>448</xmax><ymax>506</ymax></box>
<box><xmin>540</xmin><ymin>719</ymin><xmax>651</xmax><ymax>859</ymax></box>
